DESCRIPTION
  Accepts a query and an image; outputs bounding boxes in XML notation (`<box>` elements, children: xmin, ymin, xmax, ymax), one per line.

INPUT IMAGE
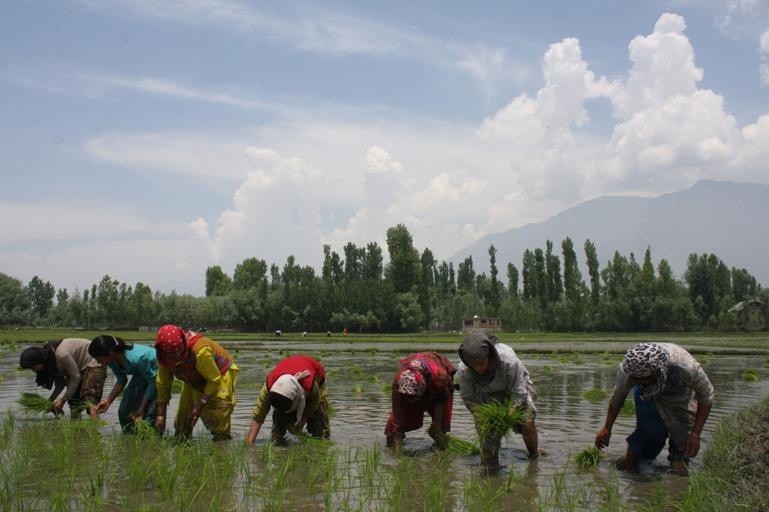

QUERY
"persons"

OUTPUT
<box><xmin>88</xmin><ymin>334</ymin><xmax>165</xmax><ymax>435</ymax></box>
<box><xmin>153</xmin><ymin>322</ymin><xmax>241</xmax><ymax>444</ymax></box>
<box><xmin>456</xmin><ymin>330</ymin><xmax>544</xmax><ymax>466</ymax></box>
<box><xmin>19</xmin><ymin>336</ymin><xmax>107</xmax><ymax>420</ymax></box>
<box><xmin>384</xmin><ymin>350</ymin><xmax>457</xmax><ymax>451</ymax></box>
<box><xmin>593</xmin><ymin>340</ymin><xmax>715</xmax><ymax>472</ymax></box>
<box><xmin>342</xmin><ymin>326</ymin><xmax>350</xmax><ymax>336</ymax></box>
<box><xmin>245</xmin><ymin>354</ymin><xmax>333</xmax><ymax>444</ymax></box>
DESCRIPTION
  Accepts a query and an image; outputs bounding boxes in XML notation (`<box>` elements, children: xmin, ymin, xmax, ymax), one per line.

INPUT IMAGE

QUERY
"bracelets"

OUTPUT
<box><xmin>104</xmin><ymin>399</ymin><xmax>111</xmax><ymax>406</ymax></box>
<box><xmin>691</xmin><ymin>432</ymin><xmax>700</xmax><ymax>438</ymax></box>
<box><xmin>155</xmin><ymin>415</ymin><xmax>164</xmax><ymax>421</ymax></box>
<box><xmin>200</xmin><ymin>398</ymin><xmax>207</xmax><ymax>404</ymax></box>
